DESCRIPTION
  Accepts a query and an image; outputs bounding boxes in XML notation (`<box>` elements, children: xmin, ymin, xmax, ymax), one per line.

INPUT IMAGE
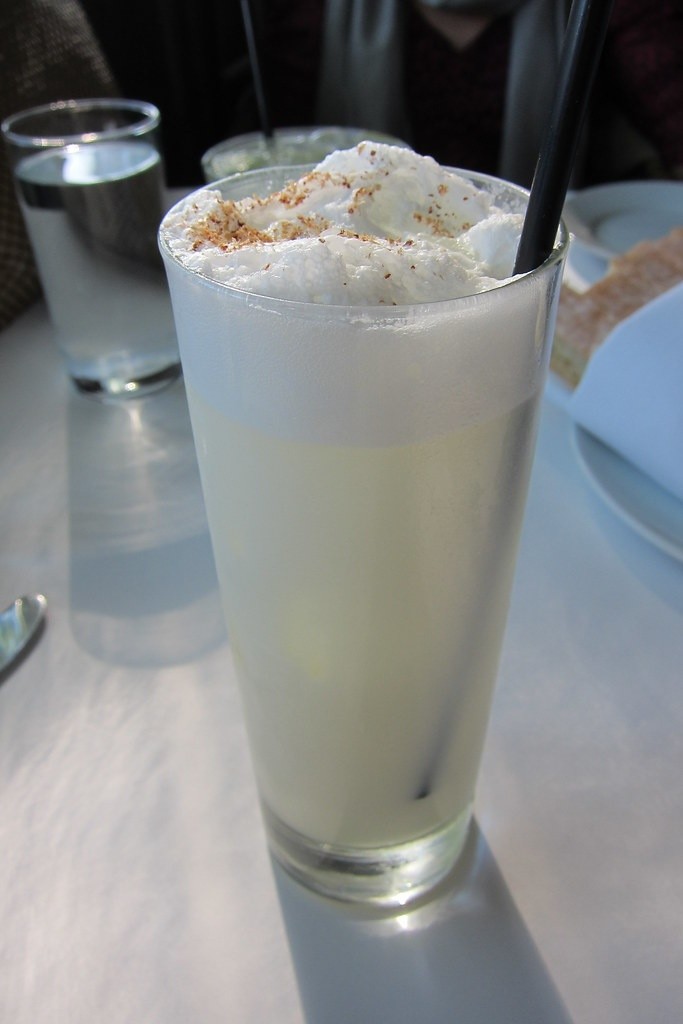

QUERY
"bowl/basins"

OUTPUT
<box><xmin>201</xmin><ymin>124</ymin><xmax>416</xmax><ymax>185</ymax></box>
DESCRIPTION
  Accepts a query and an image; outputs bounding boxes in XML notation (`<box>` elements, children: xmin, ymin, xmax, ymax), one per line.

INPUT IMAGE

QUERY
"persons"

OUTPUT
<box><xmin>0</xmin><ymin>0</ymin><xmax>111</xmax><ymax>332</ymax></box>
<box><xmin>315</xmin><ymin>0</ymin><xmax>566</xmax><ymax>187</ymax></box>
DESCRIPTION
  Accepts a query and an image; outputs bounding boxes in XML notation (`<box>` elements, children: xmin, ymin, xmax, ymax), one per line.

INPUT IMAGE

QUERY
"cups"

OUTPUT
<box><xmin>156</xmin><ymin>161</ymin><xmax>571</xmax><ymax>908</ymax></box>
<box><xmin>0</xmin><ymin>98</ymin><xmax>184</xmax><ymax>404</ymax></box>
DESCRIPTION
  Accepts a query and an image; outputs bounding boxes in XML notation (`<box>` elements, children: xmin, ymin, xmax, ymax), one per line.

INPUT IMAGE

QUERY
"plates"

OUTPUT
<box><xmin>563</xmin><ymin>181</ymin><xmax>683</xmax><ymax>263</ymax></box>
<box><xmin>571</xmin><ymin>419</ymin><xmax>682</xmax><ymax>563</ymax></box>
